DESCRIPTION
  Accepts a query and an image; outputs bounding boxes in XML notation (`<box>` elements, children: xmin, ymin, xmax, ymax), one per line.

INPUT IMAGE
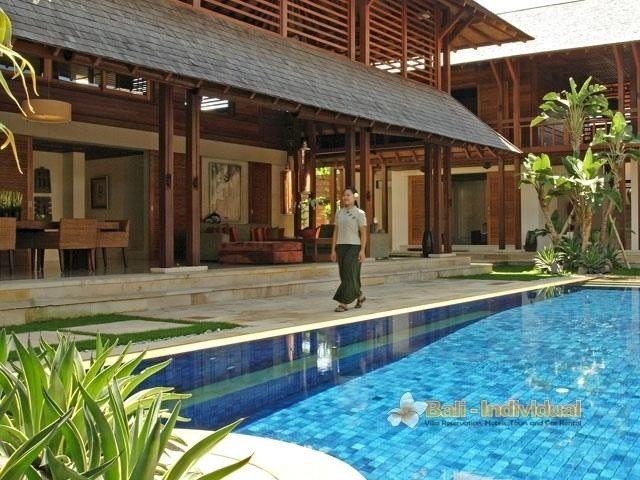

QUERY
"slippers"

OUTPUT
<box><xmin>355</xmin><ymin>296</ymin><xmax>365</xmax><ymax>308</ymax></box>
<box><xmin>334</xmin><ymin>305</ymin><xmax>347</xmax><ymax>312</ymax></box>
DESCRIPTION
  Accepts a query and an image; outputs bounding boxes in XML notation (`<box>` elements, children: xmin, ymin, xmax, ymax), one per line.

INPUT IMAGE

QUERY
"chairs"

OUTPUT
<box><xmin>305</xmin><ymin>223</ymin><xmax>334</xmax><ymax>260</ymax></box>
<box><xmin>1</xmin><ymin>216</ymin><xmax>130</xmax><ymax>275</ymax></box>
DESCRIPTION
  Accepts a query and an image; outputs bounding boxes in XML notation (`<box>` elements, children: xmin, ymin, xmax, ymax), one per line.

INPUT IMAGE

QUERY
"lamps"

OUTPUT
<box><xmin>21</xmin><ymin>45</ymin><xmax>72</xmax><ymax>124</ymax></box>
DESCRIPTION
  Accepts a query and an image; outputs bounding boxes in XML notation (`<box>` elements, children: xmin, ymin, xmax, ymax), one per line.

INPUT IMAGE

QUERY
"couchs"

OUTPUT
<box><xmin>200</xmin><ymin>222</ymin><xmax>289</xmax><ymax>260</ymax></box>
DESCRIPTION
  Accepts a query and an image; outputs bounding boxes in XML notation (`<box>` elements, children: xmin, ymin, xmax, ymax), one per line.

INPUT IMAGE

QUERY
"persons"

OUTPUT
<box><xmin>330</xmin><ymin>187</ymin><xmax>368</xmax><ymax>312</ymax></box>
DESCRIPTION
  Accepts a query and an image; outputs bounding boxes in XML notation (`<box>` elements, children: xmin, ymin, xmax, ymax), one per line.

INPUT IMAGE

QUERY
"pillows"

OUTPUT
<box><xmin>250</xmin><ymin>226</ymin><xmax>285</xmax><ymax>241</ymax></box>
<box><xmin>207</xmin><ymin>223</ymin><xmax>239</xmax><ymax>241</ymax></box>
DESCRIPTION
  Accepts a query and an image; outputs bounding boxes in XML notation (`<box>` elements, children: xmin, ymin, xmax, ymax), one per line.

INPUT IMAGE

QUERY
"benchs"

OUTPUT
<box><xmin>216</xmin><ymin>241</ymin><xmax>304</xmax><ymax>263</ymax></box>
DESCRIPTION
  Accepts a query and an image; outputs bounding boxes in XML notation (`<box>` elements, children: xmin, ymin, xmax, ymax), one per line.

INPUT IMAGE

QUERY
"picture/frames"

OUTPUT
<box><xmin>90</xmin><ymin>175</ymin><xmax>108</xmax><ymax>210</ymax></box>
